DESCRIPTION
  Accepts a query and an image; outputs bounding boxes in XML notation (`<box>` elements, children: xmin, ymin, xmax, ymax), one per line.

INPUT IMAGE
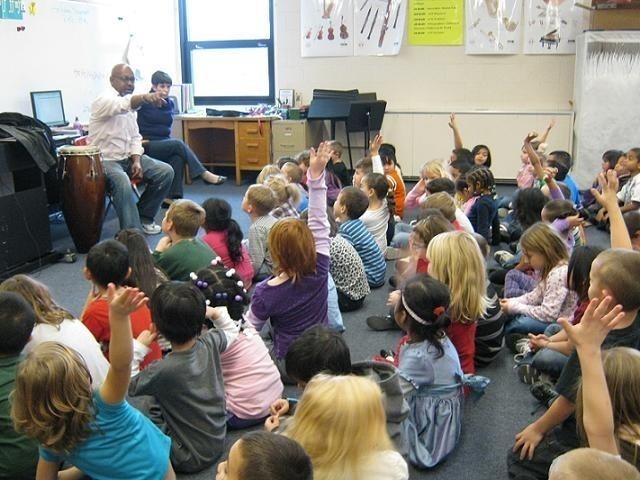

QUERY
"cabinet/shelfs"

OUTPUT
<box><xmin>272</xmin><ymin>121</ymin><xmax>321</xmax><ymax>172</ymax></box>
<box><xmin>238</xmin><ymin>121</ymin><xmax>271</xmax><ymax>172</ymax></box>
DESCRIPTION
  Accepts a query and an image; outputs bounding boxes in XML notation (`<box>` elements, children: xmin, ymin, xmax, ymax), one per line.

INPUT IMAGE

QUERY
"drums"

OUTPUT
<box><xmin>60</xmin><ymin>146</ymin><xmax>106</xmax><ymax>253</ymax></box>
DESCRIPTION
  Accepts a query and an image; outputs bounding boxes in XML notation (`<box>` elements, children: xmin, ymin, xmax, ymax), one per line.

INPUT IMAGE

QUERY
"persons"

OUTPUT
<box><xmin>138</xmin><ymin>70</ymin><xmax>227</xmax><ymax>208</ymax></box>
<box><xmin>86</xmin><ymin>62</ymin><xmax>171</xmax><ymax>238</ymax></box>
<box><xmin>0</xmin><ymin>110</ymin><xmax>640</xmax><ymax>479</ymax></box>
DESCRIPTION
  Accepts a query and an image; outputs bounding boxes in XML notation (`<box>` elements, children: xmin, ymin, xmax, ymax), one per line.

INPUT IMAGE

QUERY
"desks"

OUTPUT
<box><xmin>169</xmin><ymin>112</ymin><xmax>275</xmax><ymax>187</ymax></box>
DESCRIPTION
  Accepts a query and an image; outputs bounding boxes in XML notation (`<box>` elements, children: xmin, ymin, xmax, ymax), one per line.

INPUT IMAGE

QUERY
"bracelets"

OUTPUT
<box><xmin>140</xmin><ymin>94</ymin><xmax>150</xmax><ymax>105</ymax></box>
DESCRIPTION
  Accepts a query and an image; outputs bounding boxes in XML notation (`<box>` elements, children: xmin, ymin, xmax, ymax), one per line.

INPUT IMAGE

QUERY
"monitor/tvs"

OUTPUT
<box><xmin>29</xmin><ymin>90</ymin><xmax>66</xmax><ymax>136</ymax></box>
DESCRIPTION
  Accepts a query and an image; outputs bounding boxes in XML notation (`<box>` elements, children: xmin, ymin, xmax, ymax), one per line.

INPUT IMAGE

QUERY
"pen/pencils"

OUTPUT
<box><xmin>55</xmin><ymin>133</ymin><xmax>65</xmax><ymax>135</ymax></box>
<box><xmin>278</xmin><ymin>99</ymin><xmax>288</xmax><ymax>105</ymax></box>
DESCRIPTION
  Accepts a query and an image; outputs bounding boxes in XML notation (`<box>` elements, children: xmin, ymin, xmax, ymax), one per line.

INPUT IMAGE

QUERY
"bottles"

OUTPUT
<box><xmin>74</xmin><ymin>117</ymin><xmax>81</xmax><ymax>130</ymax></box>
<box><xmin>71</xmin><ymin>136</ymin><xmax>87</xmax><ymax>145</ymax></box>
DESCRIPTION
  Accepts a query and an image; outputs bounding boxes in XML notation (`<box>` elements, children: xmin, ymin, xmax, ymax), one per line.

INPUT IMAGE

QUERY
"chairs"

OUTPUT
<box><xmin>73</xmin><ymin>135</ymin><xmax>150</xmax><ymax>222</ymax></box>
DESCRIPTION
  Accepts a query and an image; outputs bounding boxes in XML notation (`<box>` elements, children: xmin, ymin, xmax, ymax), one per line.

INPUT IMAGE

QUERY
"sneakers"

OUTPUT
<box><xmin>141</xmin><ymin>222</ymin><xmax>161</xmax><ymax>235</ymax></box>
<box><xmin>366</xmin><ymin>315</ymin><xmax>397</xmax><ymax>330</ymax></box>
<box><xmin>506</xmin><ymin>332</ymin><xmax>558</xmax><ymax>406</ymax></box>
<box><xmin>495</xmin><ymin>251</ymin><xmax>515</xmax><ymax>266</ymax></box>
<box><xmin>497</xmin><ymin>208</ymin><xmax>509</xmax><ymax>241</ymax></box>
<box><xmin>387</xmin><ymin>247</ymin><xmax>410</xmax><ymax>290</ymax></box>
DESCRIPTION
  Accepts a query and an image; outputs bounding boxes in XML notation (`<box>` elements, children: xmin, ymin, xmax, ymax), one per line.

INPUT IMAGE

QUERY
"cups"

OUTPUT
<box><xmin>278</xmin><ymin>104</ymin><xmax>301</xmax><ymax>120</ymax></box>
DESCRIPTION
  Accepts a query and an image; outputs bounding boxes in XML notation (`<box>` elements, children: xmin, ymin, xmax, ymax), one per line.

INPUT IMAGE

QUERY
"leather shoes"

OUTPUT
<box><xmin>203</xmin><ymin>176</ymin><xmax>228</xmax><ymax>184</ymax></box>
